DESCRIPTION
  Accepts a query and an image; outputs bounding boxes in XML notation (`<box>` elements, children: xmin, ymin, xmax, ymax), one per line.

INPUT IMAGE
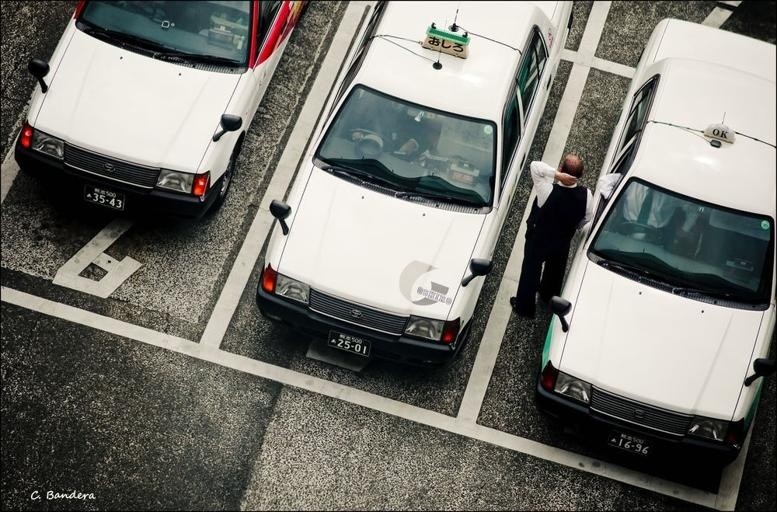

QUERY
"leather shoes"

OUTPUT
<box><xmin>511</xmin><ymin>297</ymin><xmax>518</xmax><ymax>309</ymax></box>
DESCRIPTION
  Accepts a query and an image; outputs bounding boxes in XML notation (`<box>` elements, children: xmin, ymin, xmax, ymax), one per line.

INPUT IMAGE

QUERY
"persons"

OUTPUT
<box><xmin>595</xmin><ymin>172</ymin><xmax>702</xmax><ymax>251</ymax></box>
<box><xmin>339</xmin><ymin>92</ymin><xmax>429</xmax><ymax>165</ymax></box>
<box><xmin>507</xmin><ymin>152</ymin><xmax>592</xmax><ymax>321</ymax></box>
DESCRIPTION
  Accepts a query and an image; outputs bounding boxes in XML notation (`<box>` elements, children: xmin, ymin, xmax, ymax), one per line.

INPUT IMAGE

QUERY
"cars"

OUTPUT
<box><xmin>529</xmin><ymin>13</ymin><xmax>776</xmax><ymax>469</ymax></box>
<box><xmin>12</xmin><ymin>0</ymin><xmax>313</xmax><ymax>227</ymax></box>
<box><xmin>254</xmin><ymin>0</ymin><xmax>575</xmax><ymax>371</ymax></box>
